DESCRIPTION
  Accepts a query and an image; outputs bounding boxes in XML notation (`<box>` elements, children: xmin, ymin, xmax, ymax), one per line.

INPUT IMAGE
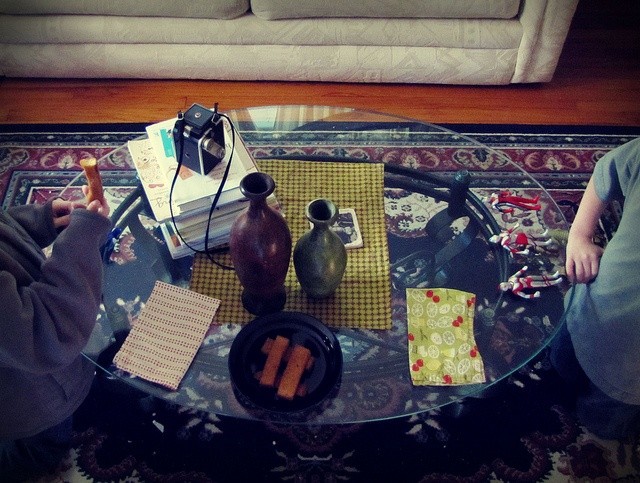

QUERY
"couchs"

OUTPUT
<box><xmin>0</xmin><ymin>0</ymin><xmax>589</xmax><ymax>84</ymax></box>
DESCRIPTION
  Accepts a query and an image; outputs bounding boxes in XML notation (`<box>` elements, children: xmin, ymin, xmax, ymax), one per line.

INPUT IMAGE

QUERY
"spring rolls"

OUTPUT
<box><xmin>277</xmin><ymin>344</ymin><xmax>311</xmax><ymax>399</ymax></box>
<box><xmin>262</xmin><ymin>338</ymin><xmax>314</xmax><ymax>369</ymax></box>
<box><xmin>259</xmin><ymin>335</ymin><xmax>290</xmax><ymax>385</ymax></box>
<box><xmin>255</xmin><ymin>371</ymin><xmax>309</xmax><ymax>397</ymax></box>
<box><xmin>80</xmin><ymin>159</ymin><xmax>104</xmax><ymax>205</ymax></box>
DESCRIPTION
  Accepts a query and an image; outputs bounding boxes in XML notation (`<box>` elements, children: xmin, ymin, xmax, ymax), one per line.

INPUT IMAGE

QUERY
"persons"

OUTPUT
<box><xmin>565</xmin><ymin>133</ymin><xmax>640</xmax><ymax>441</ymax></box>
<box><xmin>489</xmin><ymin>222</ymin><xmax>553</xmax><ymax>255</ymax></box>
<box><xmin>498</xmin><ymin>265</ymin><xmax>563</xmax><ymax>300</ymax></box>
<box><xmin>477</xmin><ymin>191</ymin><xmax>542</xmax><ymax>213</ymax></box>
<box><xmin>0</xmin><ymin>184</ymin><xmax>113</xmax><ymax>441</ymax></box>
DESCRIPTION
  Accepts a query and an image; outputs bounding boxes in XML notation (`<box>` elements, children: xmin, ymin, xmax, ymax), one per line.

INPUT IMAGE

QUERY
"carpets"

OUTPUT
<box><xmin>4</xmin><ymin>121</ymin><xmax>638</xmax><ymax>480</ymax></box>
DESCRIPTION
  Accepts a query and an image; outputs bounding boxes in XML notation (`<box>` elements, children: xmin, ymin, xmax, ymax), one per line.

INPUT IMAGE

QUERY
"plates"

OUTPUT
<box><xmin>227</xmin><ymin>310</ymin><xmax>343</xmax><ymax>412</ymax></box>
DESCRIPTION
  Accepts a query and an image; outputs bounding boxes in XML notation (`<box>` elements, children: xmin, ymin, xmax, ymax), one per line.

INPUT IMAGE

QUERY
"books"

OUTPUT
<box><xmin>127</xmin><ymin>114</ymin><xmax>288</xmax><ymax>259</ymax></box>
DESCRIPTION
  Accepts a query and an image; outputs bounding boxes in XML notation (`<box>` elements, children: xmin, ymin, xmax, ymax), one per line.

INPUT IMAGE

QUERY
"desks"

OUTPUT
<box><xmin>40</xmin><ymin>105</ymin><xmax>580</xmax><ymax>428</ymax></box>
